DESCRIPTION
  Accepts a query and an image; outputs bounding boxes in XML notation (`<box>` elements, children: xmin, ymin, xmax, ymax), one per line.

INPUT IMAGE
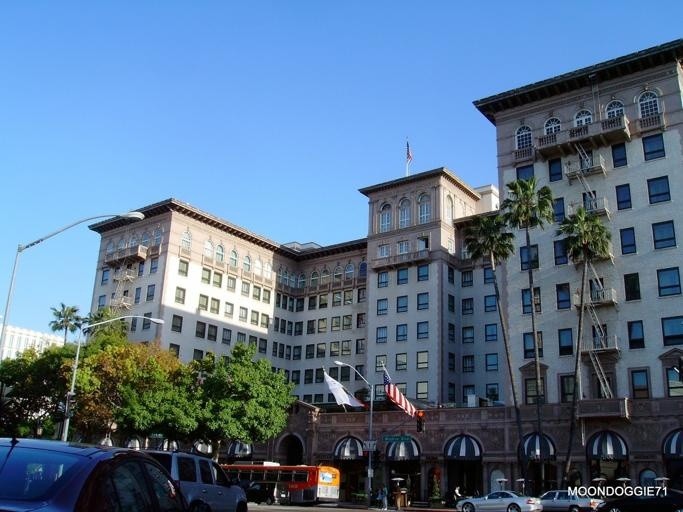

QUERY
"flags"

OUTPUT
<box><xmin>406</xmin><ymin>140</ymin><xmax>413</xmax><ymax>161</ymax></box>
<box><xmin>323</xmin><ymin>371</ymin><xmax>367</xmax><ymax>407</ymax></box>
<box><xmin>382</xmin><ymin>365</ymin><xmax>416</xmax><ymax>418</ymax></box>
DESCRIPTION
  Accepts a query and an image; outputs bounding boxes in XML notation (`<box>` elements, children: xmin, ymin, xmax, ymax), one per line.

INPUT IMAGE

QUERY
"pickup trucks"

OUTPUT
<box><xmin>540</xmin><ymin>490</ymin><xmax>606</xmax><ymax>512</ymax></box>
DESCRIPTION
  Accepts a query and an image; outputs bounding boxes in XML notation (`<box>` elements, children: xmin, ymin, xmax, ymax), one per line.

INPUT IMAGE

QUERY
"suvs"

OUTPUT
<box><xmin>244</xmin><ymin>480</ymin><xmax>290</xmax><ymax>505</ymax></box>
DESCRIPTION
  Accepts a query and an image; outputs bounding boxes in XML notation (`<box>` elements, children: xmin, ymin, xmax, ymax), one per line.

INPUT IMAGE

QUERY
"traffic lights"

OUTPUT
<box><xmin>416</xmin><ymin>410</ymin><xmax>426</xmax><ymax>433</ymax></box>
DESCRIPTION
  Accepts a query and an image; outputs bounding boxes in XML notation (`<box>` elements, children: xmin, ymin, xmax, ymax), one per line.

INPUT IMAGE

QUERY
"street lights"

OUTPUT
<box><xmin>334</xmin><ymin>360</ymin><xmax>374</xmax><ymax>507</ymax></box>
<box><xmin>61</xmin><ymin>316</ymin><xmax>165</xmax><ymax>443</ymax></box>
<box><xmin>0</xmin><ymin>211</ymin><xmax>145</xmax><ymax>365</ymax></box>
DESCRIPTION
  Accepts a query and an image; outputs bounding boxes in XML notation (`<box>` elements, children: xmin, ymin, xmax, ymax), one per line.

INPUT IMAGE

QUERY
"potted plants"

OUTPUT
<box><xmin>429</xmin><ymin>474</ymin><xmax>446</xmax><ymax>508</ymax></box>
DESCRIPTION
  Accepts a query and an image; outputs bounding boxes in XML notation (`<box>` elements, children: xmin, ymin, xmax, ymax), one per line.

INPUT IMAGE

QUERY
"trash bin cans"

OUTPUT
<box><xmin>400</xmin><ymin>491</ymin><xmax>409</xmax><ymax>508</ymax></box>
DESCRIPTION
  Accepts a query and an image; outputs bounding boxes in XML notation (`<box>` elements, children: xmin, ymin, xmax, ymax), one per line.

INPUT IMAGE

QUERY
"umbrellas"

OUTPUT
<box><xmin>516</xmin><ymin>477</ymin><xmax>532</xmax><ymax>482</ymax></box>
<box><xmin>616</xmin><ymin>477</ymin><xmax>632</xmax><ymax>482</ymax></box>
<box><xmin>391</xmin><ymin>477</ymin><xmax>405</xmax><ymax>481</ymax></box>
<box><xmin>592</xmin><ymin>477</ymin><xmax>607</xmax><ymax>482</ymax></box>
<box><xmin>496</xmin><ymin>478</ymin><xmax>509</xmax><ymax>482</ymax></box>
<box><xmin>653</xmin><ymin>475</ymin><xmax>671</xmax><ymax>480</ymax></box>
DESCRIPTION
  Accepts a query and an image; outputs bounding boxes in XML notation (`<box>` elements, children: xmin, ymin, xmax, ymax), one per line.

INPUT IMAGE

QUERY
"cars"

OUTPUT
<box><xmin>594</xmin><ymin>488</ymin><xmax>683</xmax><ymax>512</ymax></box>
<box><xmin>457</xmin><ymin>490</ymin><xmax>544</xmax><ymax>512</ymax></box>
<box><xmin>0</xmin><ymin>436</ymin><xmax>248</xmax><ymax>512</ymax></box>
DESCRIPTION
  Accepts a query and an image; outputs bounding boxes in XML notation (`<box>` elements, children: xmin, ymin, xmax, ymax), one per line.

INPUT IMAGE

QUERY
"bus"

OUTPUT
<box><xmin>218</xmin><ymin>461</ymin><xmax>341</xmax><ymax>505</ymax></box>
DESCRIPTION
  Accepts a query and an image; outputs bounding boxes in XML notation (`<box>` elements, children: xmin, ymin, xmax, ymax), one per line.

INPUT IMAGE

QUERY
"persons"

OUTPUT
<box><xmin>391</xmin><ymin>483</ymin><xmax>402</xmax><ymax>510</ymax></box>
<box><xmin>497</xmin><ymin>481</ymin><xmax>505</xmax><ymax>492</ymax></box>
<box><xmin>378</xmin><ymin>483</ymin><xmax>388</xmax><ymax>512</ymax></box>
<box><xmin>623</xmin><ymin>481</ymin><xmax>628</xmax><ymax>490</ymax></box>
<box><xmin>453</xmin><ymin>485</ymin><xmax>461</xmax><ymax>507</ymax></box>
<box><xmin>660</xmin><ymin>481</ymin><xmax>665</xmax><ymax>493</ymax></box>
<box><xmin>596</xmin><ymin>481</ymin><xmax>603</xmax><ymax>499</ymax></box>
<box><xmin>518</xmin><ymin>482</ymin><xmax>526</xmax><ymax>494</ymax></box>
